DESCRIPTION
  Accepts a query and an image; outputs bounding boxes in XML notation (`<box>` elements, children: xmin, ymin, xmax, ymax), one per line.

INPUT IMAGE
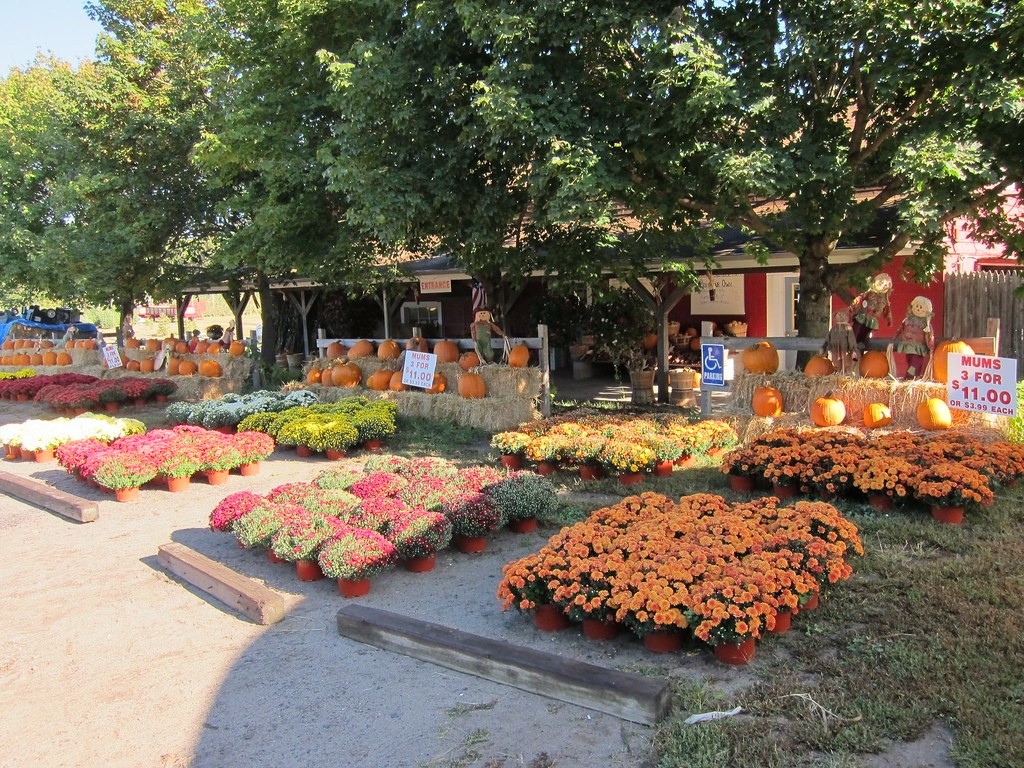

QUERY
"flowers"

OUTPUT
<box><xmin>166</xmin><ymin>389</ymin><xmax>397</xmax><ymax>453</ymax></box>
<box><xmin>55</xmin><ymin>424</ymin><xmax>276</xmax><ymax>490</ymax></box>
<box><xmin>490</xmin><ymin>413</ymin><xmax>739</xmax><ymax>474</ymax></box>
<box><xmin>208</xmin><ymin>452</ymin><xmax>560</xmax><ymax>583</ymax></box>
<box><xmin>718</xmin><ymin>426</ymin><xmax>1024</xmax><ymax>507</ymax></box>
<box><xmin>0</xmin><ymin>412</ymin><xmax>147</xmax><ymax>453</ymax></box>
<box><xmin>496</xmin><ymin>490</ymin><xmax>864</xmax><ymax>648</ymax></box>
<box><xmin>0</xmin><ymin>368</ymin><xmax>180</xmax><ymax>409</ymax></box>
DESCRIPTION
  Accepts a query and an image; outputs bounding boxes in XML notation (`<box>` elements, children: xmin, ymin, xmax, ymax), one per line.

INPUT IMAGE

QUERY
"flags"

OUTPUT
<box><xmin>472</xmin><ymin>276</ymin><xmax>488</xmax><ymax>313</ymax></box>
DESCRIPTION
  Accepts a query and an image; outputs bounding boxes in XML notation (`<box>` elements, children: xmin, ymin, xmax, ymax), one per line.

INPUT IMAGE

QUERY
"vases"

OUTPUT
<box><xmin>87</xmin><ymin>478</ymin><xmax>98</xmax><ymax>487</ymax></box>
<box><xmin>338</xmin><ymin>576</ymin><xmax>370</xmax><ymax>596</ymax></box>
<box><xmin>676</xmin><ymin>451</ymin><xmax>695</xmax><ymax>466</ymax></box>
<box><xmin>535</xmin><ymin>605</ymin><xmax>566</xmax><ymax>632</ymax></box>
<box><xmin>155</xmin><ymin>394</ymin><xmax>167</xmax><ymax>402</ymax></box>
<box><xmin>297</xmin><ymin>444</ymin><xmax>311</xmax><ymax>457</ymax></box>
<box><xmin>207</xmin><ymin>469</ymin><xmax>229</xmax><ymax>486</ymax></box>
<box><xmin>241</xmin><ymin>460</ymin><xmax>259</xmax><ymax>476</ymax></box>
<box><xmin>821</xmin><ymin>489</ymin><xmax>832</xmax><ymax>501</ymax></box>
<box><xmin>514</xmin><ymin>514</ymin><xmax>537</xmax><ymax>532</ymax></box>
<box><xmin>774</xmin><ymin>482</ymin><xmax>797</xmax><ymax>500</ymax></box>
<box><xmin>167</xmin><ymin>476</ymin><xmax>189</xmax><ymax>492</ymax></box>
<box><xmin>105</xmin><ymin>402</ymin><xmax>118</xmax><ymax>414</ymax></box>
<box><xmin>65</xmin><ymin>408</ymin><xmax>74</xmax><ymax>416</ymax></box>
<box><xmin>579</xmin><ymin>464</ymin><xmax>600</xmax><ymax>480</ymax></box>
<box><xmin>500</xmin><ymin>453</ymin><xmax>522</xmax><ymax>471</ymax></box>
<box><xmin>457</xmin><ymin>533</ymin><xmax>487</xmax><ymax>554</ymax></box>
<box><xmin>152</xmin><ymin>476</ymin><xmax>167</xmax><ymax>485</ymax></box>
<box><xmin>798</xmin><ymin>585</ymin><xmax>819</xmax><ymax>610</ymax></box>
<box><xmin>99</xmin><ymin>485</ymin><xmax>113</xmax><ymax>493</ymax></box>
<box><xmin>716</xmin><ymin>636</ymin><xmax>757</xmax><ymax>665</ymax></box>
<box><xmin>268</xmin><ymin>545</ymin><xmax>288</xmax><ymax>563</ymax></box>
<box><xmin>583</xmin><ymin>618</ymin><xmax>616</xmax><ymax>640</ymax></box>
<box><xmin>17</xmin><ymin>394</ymin><xmax>28</xmax><ymax>402</ymax></box>
<box><xmin>364</xmin><ymin>438</ymin><xmax>380</xmax><ymax>452</ymax></box>
<box><xmin>537</xmin><ymin>461</ymin><xmax>555</xmax><ymax>477</ymax></box>
<box><xmin>729</xmin><ymin>474</ymin><xmax>756</xmax><ymax>493</ymax></box>
<box><xmin>295</xmin><ymin>560</ymin><xmax>324</xmax><ymax>582</ymax></box>
<box><xmin>115</xmin><ymin>486</ymin><xmax>140</xmax><ymax>502</ymax></box>
<box><xmin>325</xmin><ymin>448</ymin><xmax>345</xmax><ymax>461</ymax></box>
<box><xmin>654</xmin><ymin>461</ymin><xmax>674</xmax><ymax>478</ymax></box>
<box><xmin>619</xmin><ymin>471</ymin><xmax>643</xmax><ymax>487</ymax></box>
<box><xmin>706</xmin><ymin>445</ymin><xmax>721</xmax><ymax>456</ymax></box>
<box><xmin>408</xmin><ymin>554</ymin><xmax>435</xmax><ymax>572</ymax></box>
<box><xmin>75</xmin><ymin>408</ymin><xmax>86</xmax><ymax>416</ymax></box>
<box><xmin>643</xmin><ymin>632</ymin><xmax>681</xmax><ymax>653</ymax></box>
<box><xmin>768</xmin><ymin>611</ymin><xmax>791</xmax><ymax>632</ymax></box>
<box><xmin>931</xmin><ymin>503</ymin><xmax>963</xmax><ymax>524</ymax></box>
<box><xmin>4</xmin><ymin>445</ymin><xmax>53</xmax><ymax>462</ymax></box>
<box><xmin>216</xmin><ymin>425</ymin><xmax>232</xmax><ymax>435</ymax></box>
<box><xmin>867</xmin><ymin>494</ymin><xmax>893</xmax><ymax>512</ymax></box>
<box><xmin>10</xmin><ymin>395</ymin><xmax>17</xmax><ymax>402</ymax></box>
<box><xmin>134</xmin><ymin>399</ymin><xmax>146</xmax><ymax>406</ymax></box>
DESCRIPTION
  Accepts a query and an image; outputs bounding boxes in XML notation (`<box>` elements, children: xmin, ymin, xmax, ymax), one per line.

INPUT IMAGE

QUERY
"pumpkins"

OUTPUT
<box><xmin>751</xmin><ymin>380</ymin><xmax>782</xmax><ymax>417</ymax></box>
<box><xmin>803</xmin><ymin>355</ymin><xmax>833</xmax><ymax>377</ymax></box>
<box><xmin>102</xmin><ymin>333</ymin><xmax>243</xmax><ymax>378</ymax></box>
<box><xmin>917</xmin><ymin>393</ymin><xmax>951</xmax><ymax>430</ymax></box>
<box><xmin>641</xmin><ymin>321</ymin><xmax>725</xmax><ymax>351</ymax></box>
<box><xmin>742</xmin><ymin>341</ymin><xmax>779</xmax><ymax>375</ymax></box>
<box><xmin>0</xmin><ymin>338</ymin><xmax>98</xmax><ymax>366</ymax></box>
<box><xmin>863</xmin><ymin>400</ymin><xmax>891</xmax><ymax>428</ymax></box>
<box><xmin>859</xmin><ymin>351</ymin><xmax>889</xmax><ymax>378</ymax></box>
<box><xmin>307</xmin><ymin>335</ymin><xmax>530</xmax><ymax>399</ymax></box>
<box><xmin>933</xmin><ymin>335</ymin><xmax>975</xmax><ymax>383</ymax></box>
<box><xmin>811</xmin><ymin>390</ymin><xmax>846</xmax><ymax>427</ymax></box>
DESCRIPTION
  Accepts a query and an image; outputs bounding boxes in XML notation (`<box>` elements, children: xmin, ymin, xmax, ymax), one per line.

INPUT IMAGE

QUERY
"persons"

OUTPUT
<box><xmin>470</xmin><ymin>304</ymin><xmax>504</xmax><ymax>364</ymax></box>
<box><xmin>818</xmin><ymin>273</ymin><xmax>894</xmax><ymax>377</ymax></box>
<box><xmin>219</xmin><ymin>320</ymin><xmax>236</xmax><ymax>352</ymax></box>
<box><xmin>61</xmin><ymin>324</ymin><xmax>77</xmax><ymax>347</ymax></box>
<box><xmin>885</xmin><ymin>296</ymin><xmax>935</xmax><ymax>381</ymax></box>
<box><xmin>122</xmin><ymin>312</ymin><xmax>136</xmax><ymax>340</ymax></box>
